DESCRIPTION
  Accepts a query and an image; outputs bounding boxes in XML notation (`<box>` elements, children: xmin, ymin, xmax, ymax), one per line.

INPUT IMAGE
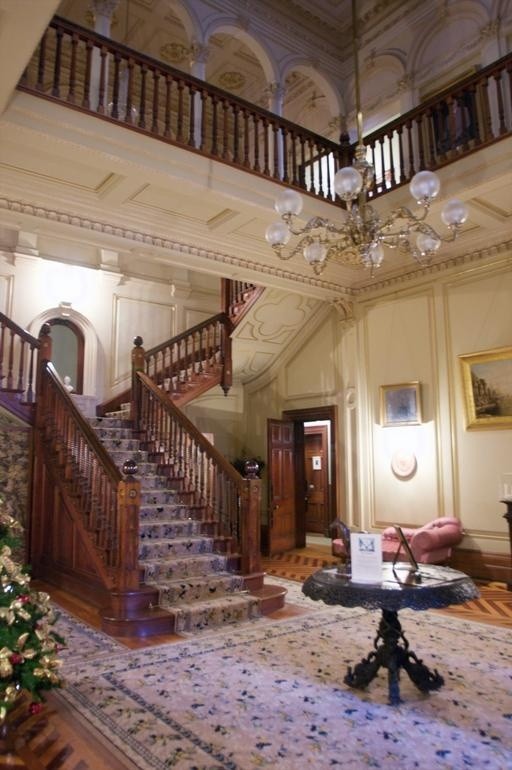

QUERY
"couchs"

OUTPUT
<box><xmin>332</xmin><ymin>516</ymin><xmax>463</xmax><ymax>564</ymax></box>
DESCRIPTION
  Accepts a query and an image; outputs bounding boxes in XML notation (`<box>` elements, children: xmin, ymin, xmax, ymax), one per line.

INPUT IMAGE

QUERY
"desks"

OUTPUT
<box><xmin>302</xmin><ymin>562</ymin><xmax>482</xmax><ymax>705</ymax></box>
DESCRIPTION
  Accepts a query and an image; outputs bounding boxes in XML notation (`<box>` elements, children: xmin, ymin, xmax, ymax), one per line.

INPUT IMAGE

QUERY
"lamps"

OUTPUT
<box><xmin>262</xmin><ymin>3</ymin><xmax>469</xmax><ymax>280</ymax></box>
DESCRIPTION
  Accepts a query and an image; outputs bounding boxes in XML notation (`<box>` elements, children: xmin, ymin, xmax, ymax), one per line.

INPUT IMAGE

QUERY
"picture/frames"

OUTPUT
<box><xmin>455</xmin><ymin>343</ymin><xmax>511</xmax><ymax>430</ymax></box>
<box><xmin>419</xmin><ymin>63</ymin><xmax>492</xmax><ymax>167</ymax></box>
<box><xmin>396</xmin><ymin>449</ymin><xmax>416</xmax><ymax>478</ymax></box>
<box><xmin>378</xmin><ymin>381</ymin><xmax>423</xmax><ymax>427</ymax></box>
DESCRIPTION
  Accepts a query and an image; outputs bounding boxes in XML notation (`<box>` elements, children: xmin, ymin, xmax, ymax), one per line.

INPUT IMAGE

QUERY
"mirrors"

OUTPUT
<box><xmin>41</xmin><ymin>317</ymin><xmax>84</xmax><ymax>395</ymax></box>
